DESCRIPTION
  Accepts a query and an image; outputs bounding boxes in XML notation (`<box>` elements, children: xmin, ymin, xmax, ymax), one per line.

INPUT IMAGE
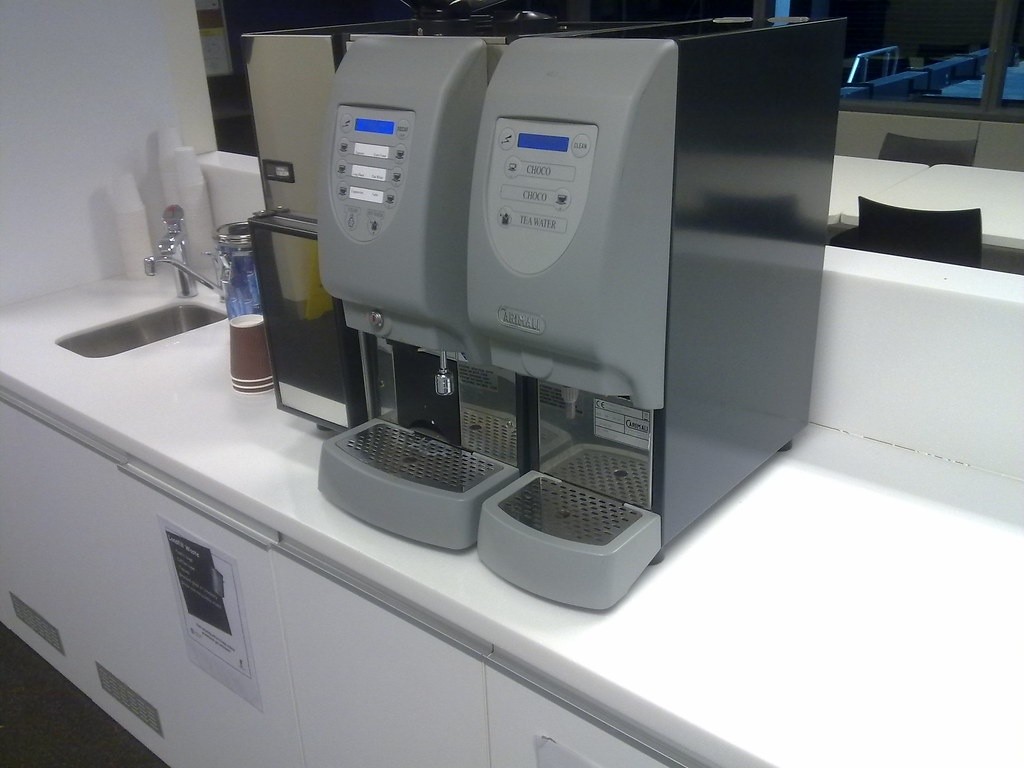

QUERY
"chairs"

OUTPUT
<box><xmin>880</xmin><ymin>131</ymin><xmax>977</xmax><ymax>165</ymax></box>
<box><xmin>857</xmin><ymin>195</ymin><xmax>981</xmax><ymax>270</ymax></box>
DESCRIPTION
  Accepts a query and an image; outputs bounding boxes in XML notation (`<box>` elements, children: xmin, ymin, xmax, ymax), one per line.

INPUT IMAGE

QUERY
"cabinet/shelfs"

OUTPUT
<box><xmin>271</xmin><ymin>550</ymin><xmax>669</xmax><ymax>768</ymax></box>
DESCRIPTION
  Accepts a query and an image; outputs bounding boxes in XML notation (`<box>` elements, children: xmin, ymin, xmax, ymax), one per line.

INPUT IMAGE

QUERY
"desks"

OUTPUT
<box><xmin>842</xmin><ymin>163</ymin><xmax>1024</xmax><ymax>249</ymax></box>
<box><xmin>828</xmin><ymin>155</ymin><xmax>928</xmax><ymax>224</ymax></box>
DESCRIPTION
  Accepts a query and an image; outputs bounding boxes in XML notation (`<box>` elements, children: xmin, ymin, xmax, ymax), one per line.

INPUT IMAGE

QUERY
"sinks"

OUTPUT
<box><xmin>56</xmin><ymin>304</ymin><xmax>228</xmax><ymax>359</ymax></box>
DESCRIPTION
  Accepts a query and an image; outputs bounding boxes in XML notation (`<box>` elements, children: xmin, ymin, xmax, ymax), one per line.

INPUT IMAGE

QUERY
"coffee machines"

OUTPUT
<box><xmin>240</xmin><ymin>10</ymin><xmax>852</xmax><ymax>611</ymax></box>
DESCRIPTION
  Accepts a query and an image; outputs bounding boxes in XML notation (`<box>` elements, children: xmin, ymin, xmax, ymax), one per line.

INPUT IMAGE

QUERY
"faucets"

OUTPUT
<box><xmin>157</xmin><ymin>204</ymin><xmax>199</xmax><ymax>299</ymax></box>
<box><xmin>143</xmin><ymin>246</ymin><xmax>227</xmax><ymax>304</ymax></box>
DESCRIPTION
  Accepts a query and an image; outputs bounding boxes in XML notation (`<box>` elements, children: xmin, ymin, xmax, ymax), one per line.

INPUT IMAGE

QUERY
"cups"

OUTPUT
<box><xmin>112</xmin><ymin>172</ymin><xmax>158</xmax><ymax>280</ymax></box>
<box><xmin>230</xmin><ymin>314</ymin><xmax>274</xmax><ymax>394</ymax></box>
<box><xmin>158</xmin><ymin>126</ymin><xmax>218</xmax><ymax>278</ymax></box>
